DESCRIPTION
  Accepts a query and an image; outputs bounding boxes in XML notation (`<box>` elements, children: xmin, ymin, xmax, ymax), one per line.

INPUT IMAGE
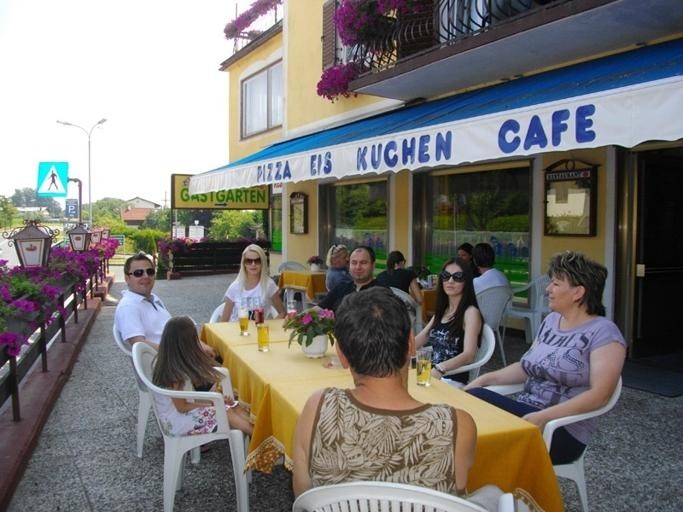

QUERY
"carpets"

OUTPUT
<box><xmin>618</xmin><ymin>358</ymin><xmax>682</xmax><ymax>401</ymax></box>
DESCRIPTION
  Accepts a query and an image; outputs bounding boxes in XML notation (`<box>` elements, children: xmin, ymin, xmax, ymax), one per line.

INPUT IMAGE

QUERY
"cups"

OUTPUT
<box><xmin>287</xmin><ymin>301</ymin><xmax>298</xmax><ymax>319</ymax></box>
<box><xmin>417</xmin><ymin>274</ymin><xmax>439</xmax><ymax>290</ymax></box>
<box><xmin>415</xmin><ymin>349</ymin><xmax>433</xmax><ymax>385</ymax></box>
<box><xmin>236</xmin><ymin>297</ymin><xmax>271</xmax><ymax>352</ymax></box>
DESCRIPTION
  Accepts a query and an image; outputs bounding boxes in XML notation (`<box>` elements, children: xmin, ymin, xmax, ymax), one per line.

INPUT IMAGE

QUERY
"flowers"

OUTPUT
<box><xmin>305</xmin><ymin>254</ymin><xmax>325</xmax><ymax>267</ymax></box>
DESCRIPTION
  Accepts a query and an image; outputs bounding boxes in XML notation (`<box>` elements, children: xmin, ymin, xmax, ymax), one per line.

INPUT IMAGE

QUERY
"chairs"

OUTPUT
<box><xmin>112</xmin><ymin>321</ymin><xmax>201</xmax><ymax>464</ymax></box>
<box><xmin>267</xmin><ymin>260</ymin><xmax>556</xmax><ymax>390</ymax></box>
<box><xmin>209</xmin><ymin>302</ymin><xmax>245</xmax><ymax>323</ymax></box>
<box><xmin>131</xmin><ymin>342</ymin><xmax>253</xmax><ymax>511</ymax></box>
<box><xmin>296</xmin><ymin>481</ymin><xmax>515</xmax><ymax>511</ymax></box>
<box><xmin>482</xmin><ymin>360</ymin><xmax>623</xmax><ymax>510</ymax></box>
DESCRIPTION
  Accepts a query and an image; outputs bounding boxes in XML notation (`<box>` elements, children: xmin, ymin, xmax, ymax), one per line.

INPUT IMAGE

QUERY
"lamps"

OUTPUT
<box><xmin>0</xmin><ymin>216</ymin><xmax>112</xmax><ymax>269</ymax></box>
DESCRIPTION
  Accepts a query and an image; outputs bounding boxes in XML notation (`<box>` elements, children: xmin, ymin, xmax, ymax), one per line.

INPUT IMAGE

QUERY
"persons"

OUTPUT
<box><xmin>463</xmin><ymin>254</ymin><xmax>625</xmax><ymax>467</ymax></box>
<box><xmin>220</xmin><ymin>245</ymin><xmax>287</xmax><ymax>323</ymax></box>
<box><xmin>151</xmin><ymin>316</ymin><xmax>256</xmax><ymax>439</ymax></box>
<box><xmin>319</xmin><ymin>242</ymin><xmax>512</xmax><ymax>387</ymax></box>
<box><xmin>290</xmin><ymin>289</ymin><xmax>508</xmax><ymax>512</ymax></box>
<box><xmin>117</xmin><ymin>255</ymin><xmax>221</xmax><ymax>393</ymax></box>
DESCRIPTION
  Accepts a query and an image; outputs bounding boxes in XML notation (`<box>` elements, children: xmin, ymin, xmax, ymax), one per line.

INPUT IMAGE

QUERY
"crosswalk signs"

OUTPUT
<box><xmin>35</xmin><ymin>161</ymin><xmax>69</xmax><ymax>197</ymax></box>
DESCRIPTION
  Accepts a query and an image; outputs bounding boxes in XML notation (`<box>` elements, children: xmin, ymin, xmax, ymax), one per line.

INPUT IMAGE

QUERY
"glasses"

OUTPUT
<box><xmin>440</xmin><ymin>270</ymin><xmax>466</xmax><ymax>283</ymax></box>
<box><xmin>126</xmin><ymin>268</ymin><xmax>155</xmax><ymax>278</ymax></box>
<box><xmin>242</xmin><ymin>256</ymin><xmax>262</xmax><ymax>265</ymax></box>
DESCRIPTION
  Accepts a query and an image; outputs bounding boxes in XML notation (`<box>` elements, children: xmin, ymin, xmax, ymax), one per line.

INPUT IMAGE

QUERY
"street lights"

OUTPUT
<box><xmin>55</xmin><ymin>116</ymin><xmax>111</xmax><ymax>231</ymax></box>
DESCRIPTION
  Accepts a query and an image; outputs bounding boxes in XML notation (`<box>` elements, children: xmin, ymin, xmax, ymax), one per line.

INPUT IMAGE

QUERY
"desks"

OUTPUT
<box><xmin>201</xmin><ymin>318</ymin><xmax>565</xmax><ymax>511</ymax></box>
